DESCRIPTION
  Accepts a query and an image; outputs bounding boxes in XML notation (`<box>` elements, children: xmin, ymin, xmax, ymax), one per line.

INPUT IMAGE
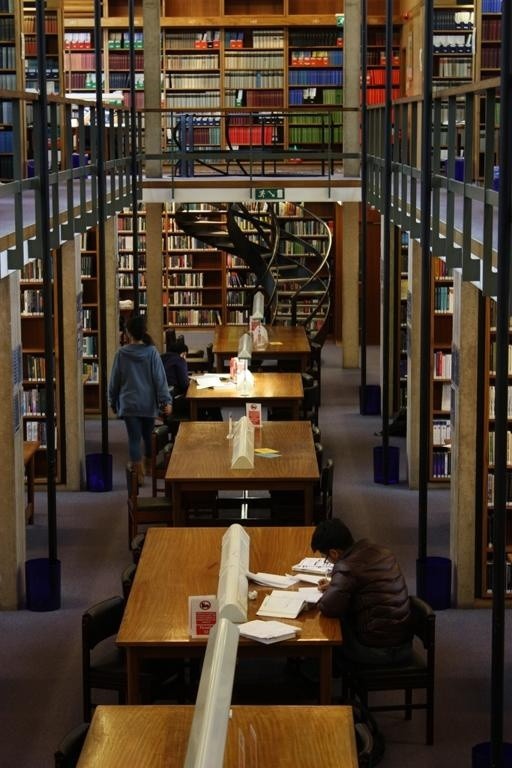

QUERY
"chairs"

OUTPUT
<box><xmin>302</xmin><ymin>372</ymin><xmax>313</xmax><ymax>387</ymax></box>
<box><xmin>54</xmin><ymin>723</ymin><xmax>90</xmax><ymax>767</ymax></box>
<box><xmin>166</xmin><ymin>327</ymin><xmax>230</xmax><ymax>372</ymax></box>
<box><xmin>122</xmin><ymin>564</ymin><xmax>137</xmax><ymax>601</ymax></box>
<box><xmin>151</xmin><ymin>425</ymin><xmax>220</xmax><ymax>518</ymax></box>
<box><xmin>312</xmin><ymin>424</ymin><xmax>320</xmax><ymax>442</ymax></box>
<box><xmin>268</xmin><ymin>379</ymin><xmax>321</xmax><ymax>426</ymax></box>
<box><xmin>269</xmin><ymin>458</ymin><xmax>334</xmax><ymax>525</ymax></box>
<box><xmin>131</xmin><ymin>533</ymin><xmax>145</xmax><ymax>564</ymax></box>
<box><xmin>126</xmin><ymin>461</ymin><xmax>186</xmax><ymax>552</ymax></box>
<box><xmin>315</xmin><ymin>442</ymin><xmax>323</xmax><ymax>473</ymax></box>
<box><xmin>349</xmin><ymin>596</ymin><xmax>435</xmax><ymax>745</ymax></box>
<box><xmin>354</xmin><ymin>722</ymin><xmax>374</xmax><ymax>768</ymax></box>
<box><xmin>277</xmin><ymin>328</ymin><xmax>327</xmax><ymax>384</ymax></box>
<box><xmin>82</xmin><ymin>596</ymin><xmax>185</xmax><ymax>723</ymax></box>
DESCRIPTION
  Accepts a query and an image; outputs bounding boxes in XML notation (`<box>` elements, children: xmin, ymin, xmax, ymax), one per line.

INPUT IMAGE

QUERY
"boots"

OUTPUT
<box><xmin>130</xmin><ymin>456</ymin><xmax>157</xmax><ymax>487</ymax></box>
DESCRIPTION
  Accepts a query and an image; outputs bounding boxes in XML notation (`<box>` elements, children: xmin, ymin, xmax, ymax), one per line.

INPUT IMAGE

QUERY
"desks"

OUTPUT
<box><xmin>185</xmin><ymin>373</ymin><xmax>305</xmax><ymax>421</ymax></box>
<box><xmin>212</xmin><ymin>325</ymin><xmax>312</xmax><ymax>373</ymax></box>
<box><xmin>165</xmin><ymin>421</ymin><xmax>321</xmax><ymax>526</ymax></box>
<box><xmin>115</xmin><ymin>526</ymin><xmax>344</xmax><ymax>704</ymax></box>
<box><xmin>76</xmin><ymin>705</ymin><xmax>358</xmax><ymax>768</ymax></box>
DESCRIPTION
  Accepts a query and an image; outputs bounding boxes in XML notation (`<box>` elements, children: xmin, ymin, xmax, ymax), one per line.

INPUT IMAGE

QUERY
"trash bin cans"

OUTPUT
<box><xmin>416</xmin><ymin>557</ymin><xmax>451</xmax><ymax>610</ymax></box>
<box><xmin>473</xmin><ymin>742</ymin><xmax>512</xmax><ymax>768</ymax></box>
<box><xmin>373</xmin><ymin>447</ymin><xmax>399</xmax><ymax>484</ymax></box>
<box><xmin>87</xmin><ymin>453</ymin><xmax>113</xmax><ymax>491</ymax></box>
<box><xmin>360</xmin><ymin>385</ymin><xmax>380</xmax><ymax>414</ymax></box>
<box><xmin>26</xmin><ymin>558</ymin><xmax>61</xmax><ymax>611</ymax></box>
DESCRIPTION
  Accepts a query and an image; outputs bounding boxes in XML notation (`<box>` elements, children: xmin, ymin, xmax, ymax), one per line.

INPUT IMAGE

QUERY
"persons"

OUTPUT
<box><xmin>110</xmin><ymin>317</ymin><xmax>174</xmax><ymax>486</ymax></box>
<box><xmin>312</xmin><ymin>517</ymin><xmax>413</xmax><ymax>668</ymax></box>
<box><xmin>159</xmin><ymin>340</ymin><xmax>189</xmax><ymax>396</ymax></box>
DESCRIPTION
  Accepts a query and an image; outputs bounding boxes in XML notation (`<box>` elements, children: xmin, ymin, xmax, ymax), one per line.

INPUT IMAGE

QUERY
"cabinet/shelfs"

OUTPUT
<box><xmin>5</xmin><ymin>2</ymin><xmax>27</xmax><ymax>183</ymax></box>
<box><xmin>380</xmin><ymin>214</ymin><xmax>407</xmax><ymax>418</ymax></box>
<box><xmin>480</xmin><ymin>5</ymin><xmax>503</xmax><ymax>180</ymax></box>
<box><xmin>81</xmin><ymin>215</ymin><xmax>120</xmax><ymax>421</ymax></box>
<box><xmin>21</xmin><ymin>234</ymin><xmax>88</xmax><ymax>491</ymax></box>
<box><xmin>62</xmin><ymin>22</ymin><xmax>402</xmax><ymax>168</ymax></box>
<box><xmin>0</xmin><ymin>269</ymin><xmax>28</xmax><ymax>611</ymax></box>
<box><xmin>24</xmin><ymin>8</ymin><xmax>64</xmax><ymax>172</ymax></box>
<box><xmin>450</xmin><ymin>268</ymin><xmax>512</xmax><ymax>610</ymax></box>
<box><xmin>406</xmin><ymin>238</ymin><xmax>452</xmax><ymax>490</ymax></box>
<box><xmin>428</xmin><ymin>5</ymin><xmax>475</xmax><ymax>177</ymax></box>
<box><xmin>118</xmin><ymin>202</ymin><xmax>336</xmax><ymax>344</ymax></box>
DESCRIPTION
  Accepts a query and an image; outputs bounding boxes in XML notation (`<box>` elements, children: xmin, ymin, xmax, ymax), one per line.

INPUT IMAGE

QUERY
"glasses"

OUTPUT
<box><xmin>324</xmin><ymin>554</ymin><xmax>329</xmax><ymax>563</ymax></box>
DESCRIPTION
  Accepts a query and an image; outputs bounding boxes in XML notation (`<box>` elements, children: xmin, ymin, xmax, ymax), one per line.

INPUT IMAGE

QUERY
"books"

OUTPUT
<box><xmin>488</xmin><ymin>302</ymin><xmax>512</xmax><ymax>592</ymax></box>
<box><xmin>241</xmin><ymin>557</ymin><xmax>334</xmax><ymax>645</ymax></box>
<box><xmin>118</xmin><ymin>198</ymin><xmax>335</xmax><ymax>337</ymax></box>
<box><xmin>0</xmin><ymin>0</ymin><xmax>500</xmax><ymax>191</ymax></box>
<box><xmin>17</xmin><ymin>262</ymin><xmax>57</xmax><ymax>452</ymax></box>
<box><xmin>433</xmin><ymin>256</ymin><xmax>455</xmax><ymax>478</ymax></box>
<box><xmin>77</xmin><ymin>234</ymin><xmax>98</xmax><ymax>385</ymax></box>
<box><xmin>395</xmin><ymin>233</ymin><xmax>410</xmax><ymax>413</ymax></box>
<box><xmin>190</xmin><ymin>372</ymin><xmax>232</xmax><ymax>391</ymax></box>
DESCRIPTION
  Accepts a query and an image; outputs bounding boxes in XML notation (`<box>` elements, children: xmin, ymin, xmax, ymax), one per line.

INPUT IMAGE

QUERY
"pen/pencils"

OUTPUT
<box><xmin>325</xmin><ymin>571</ymin><xmax>328</xmax><ymax>584</ymax></box>
